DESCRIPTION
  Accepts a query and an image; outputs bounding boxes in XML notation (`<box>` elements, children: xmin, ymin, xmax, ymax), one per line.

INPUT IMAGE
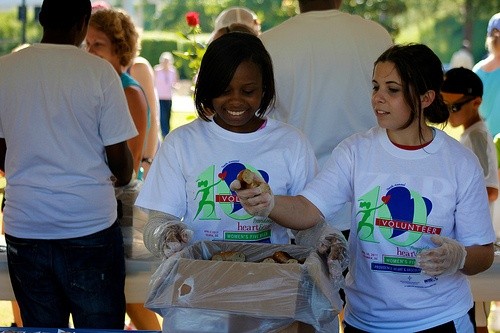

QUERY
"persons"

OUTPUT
<box><xmin>471</xmin><ymin>12</ymin><xmax>500</xmax><ymax>138</ymax></box>
<box><xmin>155</xmin><ymin>53</ymin><xmax>177</xmax><ymax>136</ymax></box>
<box><xmin>0</xmin><ymin>0</ymin><xmax>139</xmax><ymax>331</ymax></box>
<box><xmin>85</xmin><ymin>10</ymin><xmax>161</xmax><ymax>331</ymax></box>
<box><xmin>259</xmin><ymin>0</ymin><xmax>395</xmax><ymax>332</ymax></box>
<box><xmin>440</xmin><ymin>67</ymin><xmax>498</xmax><ymax>333</ymax></box>
<box><xmin>230</xmin><ymin>44</ymin><xmax>495</xmax><ymax>333</ymax></box>
<box><xmin>208</xmin><ymin>9</ymin><xmax>260</xmax><ymax>40</ymax></box>
<box><xmin>132</xmin><ymin>33</ymin><xmax>349</xmax><ymax>271</ymax></box>
<box><xmin>90</xmin><ymin>5</ymin><xmax>160</xmax><ymax>180</ymax></box>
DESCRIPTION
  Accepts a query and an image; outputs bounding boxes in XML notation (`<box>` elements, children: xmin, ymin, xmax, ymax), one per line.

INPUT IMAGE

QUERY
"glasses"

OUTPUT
<box><xmin>448</xmin><ymin>97</ymin><xmax>476</xmax><ymax>114</ymax></box>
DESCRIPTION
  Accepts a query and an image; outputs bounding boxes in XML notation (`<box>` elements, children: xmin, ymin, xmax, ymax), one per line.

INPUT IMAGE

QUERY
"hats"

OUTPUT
<box><xmin>487</xmin><ymin>13</ymin><xmax>500</xmax><ymax>33</ymax></box>
<box><xmin>205</xmin><ymin>6</ymin><xmax>261</xmax><ymax>45</ymax></box>
<box><xmin>440</xmin><ymin>65</ymin><xmax>484</xmax><ymax>97</ymax></box>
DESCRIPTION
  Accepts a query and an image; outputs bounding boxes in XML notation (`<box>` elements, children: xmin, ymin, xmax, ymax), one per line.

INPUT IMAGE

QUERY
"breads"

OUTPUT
<box><xmin>237</xmin><ymin>169</ymin><xmax>272</xmax><ymax>196</ymax></box>
<box><xmin>212</xmin><ymin>251</ymin><xmax>306</xmax><ymax>264</ymax></box>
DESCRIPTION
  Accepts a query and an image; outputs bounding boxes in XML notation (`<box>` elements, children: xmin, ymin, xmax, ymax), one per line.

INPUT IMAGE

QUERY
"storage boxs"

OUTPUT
<box><xmin>144</xmin><ymin>240</ymin><xmax>344</xmax><ymax>332</ymax></box>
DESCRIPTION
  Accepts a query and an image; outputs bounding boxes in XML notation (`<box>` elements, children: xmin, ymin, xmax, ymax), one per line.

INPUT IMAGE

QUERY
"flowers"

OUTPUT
<box><xmin>172</xmin><ymin>12</ymin><xmax>206</xmax><ymax>73</ymax></box>
